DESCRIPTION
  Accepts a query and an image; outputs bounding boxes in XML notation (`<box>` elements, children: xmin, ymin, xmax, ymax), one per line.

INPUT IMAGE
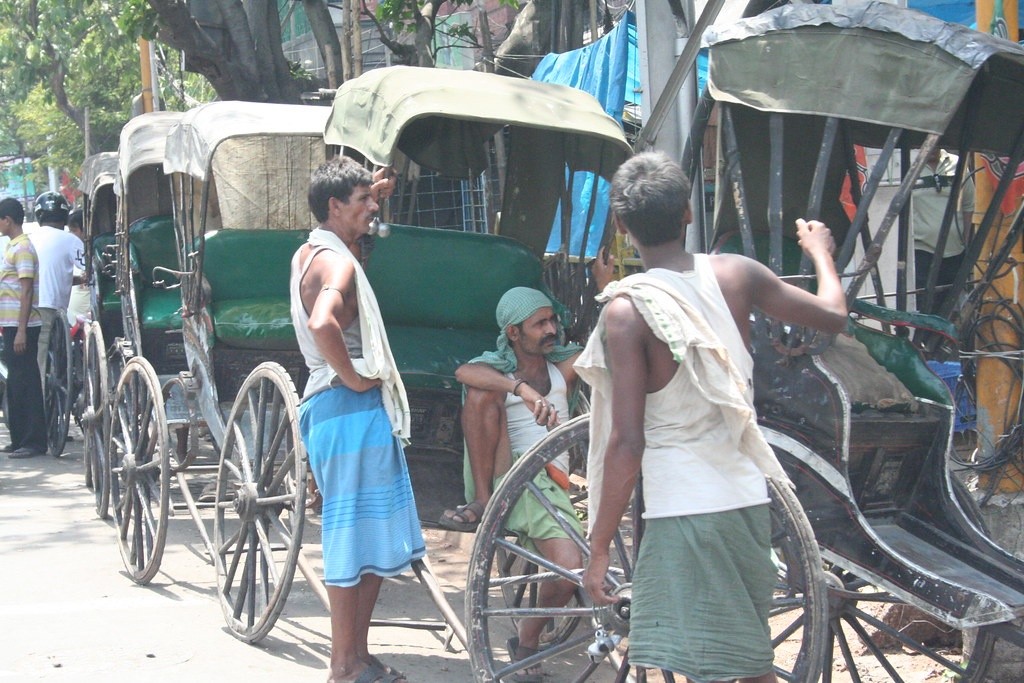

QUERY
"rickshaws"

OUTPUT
<box><xmin>461</xmin><ymin>0</ymin><xmax>1024</xmax><ymax>683</ymax></box>
<box><xmin>211</xmin><ymin>64</ymin><xmax>639</xmax><ymax>683</ymax></box>
<box><xmin>40</xmin><ymin>109</ymin><xmax>219</xmax><ymax>585</ymax></box>
<box><xmin>105</xmin><ymin>102</ymin><xmax>467</xmax><ymax>654</ymax></box>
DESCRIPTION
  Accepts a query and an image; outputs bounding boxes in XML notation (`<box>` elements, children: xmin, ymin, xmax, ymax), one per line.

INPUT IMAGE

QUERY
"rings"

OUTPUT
<box><xmin>534</xmin><ymin>399</ymin><xmax>555</xmax><ymax>415</ymax></box>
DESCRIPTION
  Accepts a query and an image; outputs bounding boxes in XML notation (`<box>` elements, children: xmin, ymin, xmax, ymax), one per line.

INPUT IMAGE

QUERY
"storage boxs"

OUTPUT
<box><xmin>926</xmin><ymin>359</ymin><xmax>978</xmax><ymax>431</ymax></box>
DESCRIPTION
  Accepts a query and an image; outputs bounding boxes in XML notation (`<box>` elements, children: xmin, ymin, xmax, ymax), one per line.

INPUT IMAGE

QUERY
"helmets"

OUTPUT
<box><xmin>34</xmin><ymin>191</ymin><xmax>71</xmax><ymax>225</ymax></box>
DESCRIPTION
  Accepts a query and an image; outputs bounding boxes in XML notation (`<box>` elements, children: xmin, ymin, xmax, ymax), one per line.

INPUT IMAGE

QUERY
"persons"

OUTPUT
<box><xmin>581</xmin><ymin>152</ymin><xmax>851</xmax><ymax>683</ymax></box>
<box><xmin>0</xmin><ymin>191</ymin><xmax>92</xmax><ymax>458</ymax></box>
<box><xmin>912</xmin><ymin>139</ymin><xmax>976</xmax><ymax>355</ymax></box>
<box><xmin>288</xmin><ymin>152</ymin><xmax>425</xmax><ymax>683</ymax></box>
<box><xmin>437</xmin><ymin>244</ymin><xmax>617</xmax><ymax>683</ymax></box>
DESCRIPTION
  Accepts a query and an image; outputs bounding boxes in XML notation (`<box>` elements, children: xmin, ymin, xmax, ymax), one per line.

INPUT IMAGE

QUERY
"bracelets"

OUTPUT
<box><xmin>510</xmin><ymin>378</ymin><xmax>530</xmax><ymax>397</ymax></box>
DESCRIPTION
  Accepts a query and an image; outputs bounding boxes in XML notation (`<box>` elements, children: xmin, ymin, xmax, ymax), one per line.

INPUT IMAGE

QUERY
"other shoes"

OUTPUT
<box><xmin>9</xmin><ymin>448</ymin><xmax>45</xmax><ymax>458</ymax></box>
<box><xmin>0</xmin><ymin>442</ymin><xmax>17</xmax><ymax>452</ymax></box>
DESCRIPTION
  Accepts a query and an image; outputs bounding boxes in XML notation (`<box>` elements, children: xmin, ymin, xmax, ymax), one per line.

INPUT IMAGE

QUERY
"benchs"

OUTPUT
<box><xmin>127</xmin><ymin>213</ymin><xmax>183</xmax><ymax>337</ymax></box>
<box><xmin>361</xmin><ymin>222</ymin><xmax>572</xmax><ymax>390</ymax></box>
<box><xmin>91</xmin><ymin>231</ymin><xmax>122</xmax><ymax>313</ymax></box>
<box><xmin>181</xmin><ymin>229</ymin><xmax>314</xmax><ymax>351</ymax></box>
<box><xmin>710</xmin><ymin>228</ymin><xmax>957</xmax><ymax>518</ymax></box>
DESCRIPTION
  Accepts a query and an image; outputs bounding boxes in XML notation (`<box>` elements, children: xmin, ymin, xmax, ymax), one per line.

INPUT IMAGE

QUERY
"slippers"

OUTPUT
<box><xmin>439</xmin><ymin>502</ymin><xmax>485</xmax><ymax>532</ymax></box>
<box><xmin>355</xmin><ymin>655</ymin><xmax>406</xmax><ymax>683</ymax></box>
<box><xmin>507</xmin><ymin>636</ymin><xmax>543</xmax><ymax>683</ymax></box>
